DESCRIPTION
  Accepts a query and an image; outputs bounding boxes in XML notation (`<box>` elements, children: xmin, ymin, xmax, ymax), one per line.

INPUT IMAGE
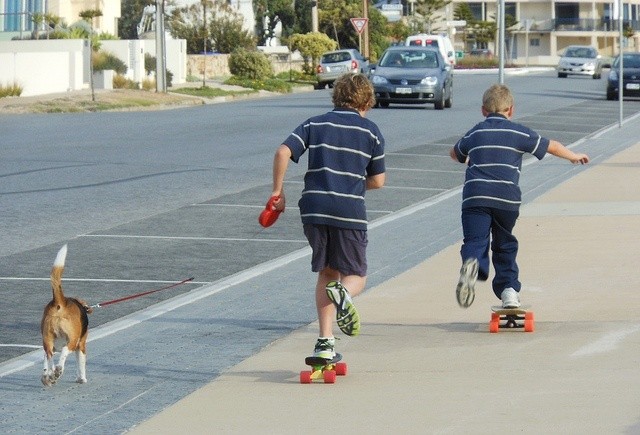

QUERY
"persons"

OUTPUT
<box><xmin>449</xmin><ymin>85</ymin><xmax>589</xmax><ymax>308</ymax></box>
<box><xmin>269</xmin><ymin>72</ymin><xmax>386</xmax><ymax>360</ymax></box>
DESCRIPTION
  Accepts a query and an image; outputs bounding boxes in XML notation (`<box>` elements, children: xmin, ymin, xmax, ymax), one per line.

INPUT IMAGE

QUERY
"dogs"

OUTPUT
<box><xmin>40</xmin><ymin>241</ymin><xmax>93</xmax><ymax>386</ymax></box>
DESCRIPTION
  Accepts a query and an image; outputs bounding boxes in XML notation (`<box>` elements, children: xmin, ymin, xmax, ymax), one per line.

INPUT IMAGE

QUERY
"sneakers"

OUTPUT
<box><xmin>314</xmin><ymin>338</ymin><xmax>336</xmax><ymax>360</ymax></box>
<box><xmin>501</xmin><ymin>288</ymin><xmax>520</xmax><ymax>309</ymax></box>
<box><xmin>325</xmin><ymin>281</ymin><xmax>360</xmax><ymax>336</ymax></box>
<box><xmin>457</xmin><ymin>258</ymin><xmax>479</xmax><ymax>308</ymax></box>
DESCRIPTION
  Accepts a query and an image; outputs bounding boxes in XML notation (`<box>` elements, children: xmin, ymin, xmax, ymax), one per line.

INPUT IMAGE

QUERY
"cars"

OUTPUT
<box><xmin>372</xmin><ymin>45</ymin><xmax>454</xmax><ymax>110</ymax></box>
<box><xmin>317</xmin><ymin>48</ymin><xmax>366</xmax><ymax>87</ymax></box>
<box><xmin>556</xmin><ymin>44</ymin><xmax>603</xmax><ymax>79</ymax></box>
<box><xmin>601</xmin><ymin>51</ymin><xmax>640</xmax><ymax>100</ymax></box>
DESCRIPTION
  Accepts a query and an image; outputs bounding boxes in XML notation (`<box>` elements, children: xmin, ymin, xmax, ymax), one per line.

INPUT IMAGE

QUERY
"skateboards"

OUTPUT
<box><xmin>489</xmin><ymin>305</ymin><xmax>535</xmax><ymax>332</ymax></box>
<box><xmin>300</xmin><ymin>353</ymin><xmax>347</xmax><ymax>383</ymax></box>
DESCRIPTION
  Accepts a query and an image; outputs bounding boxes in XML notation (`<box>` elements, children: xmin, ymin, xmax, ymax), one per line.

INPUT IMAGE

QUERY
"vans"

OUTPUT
<box><xmin>406</xmin><ymin>33</ymin><xmax>456</xmax><ymax>70</ymax></box>
